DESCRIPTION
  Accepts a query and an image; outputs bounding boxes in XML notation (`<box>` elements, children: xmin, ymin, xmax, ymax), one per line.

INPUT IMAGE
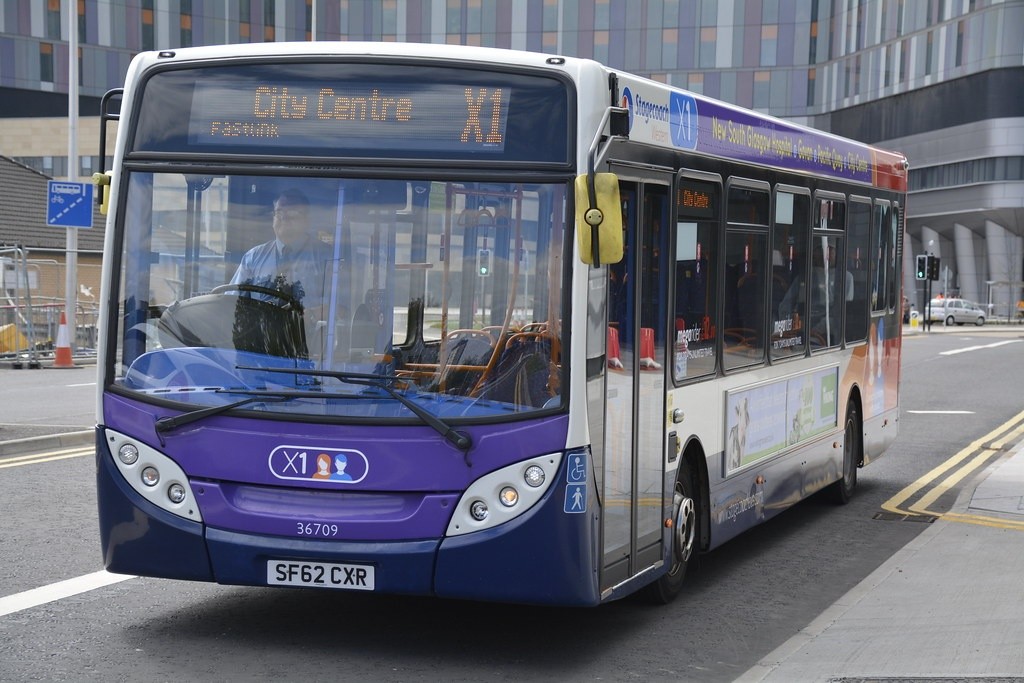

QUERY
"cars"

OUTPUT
<box><xmin>925</xmin><ymin>298</ymin><xmax>986</xmax><ymax>326</ymax></box>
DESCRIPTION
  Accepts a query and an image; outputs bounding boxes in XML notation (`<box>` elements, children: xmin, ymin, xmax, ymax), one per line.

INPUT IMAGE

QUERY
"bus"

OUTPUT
<box><xmin>95</xmin><ymin>42</ymin><xmax>908</xmax><ymax>611</ymax></box>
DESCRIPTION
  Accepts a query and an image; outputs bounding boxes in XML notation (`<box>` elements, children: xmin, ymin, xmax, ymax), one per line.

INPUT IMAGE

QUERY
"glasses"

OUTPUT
<box><xmin>270</xmin><ymin>208</ymin><xmax>304</xmax><ymax>221</ymax></box>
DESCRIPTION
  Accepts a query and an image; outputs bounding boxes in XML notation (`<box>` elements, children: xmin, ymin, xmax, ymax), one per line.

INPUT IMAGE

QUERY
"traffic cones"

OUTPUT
<box><xmin>42</xmin><ymin>311</ymin><xmax>83</xmax><ymax>368</ymax></box>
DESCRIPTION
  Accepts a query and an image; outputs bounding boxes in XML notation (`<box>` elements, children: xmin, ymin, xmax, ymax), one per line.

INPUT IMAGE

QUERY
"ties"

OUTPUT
<box><xmin>278</xmin><ymin>246</ymin><xmax>291</xmax><ymax>273</ymax></box>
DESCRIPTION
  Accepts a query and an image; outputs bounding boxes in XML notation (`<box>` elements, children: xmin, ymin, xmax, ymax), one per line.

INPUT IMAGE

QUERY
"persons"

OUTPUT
<box><xmin>904</xmin><ymin>299</ymin><xmax>910</xmax><ymax>324</ymax></box>
<box><xmin>777</xmin><ymin>239</ymin><xmax>855</xmax><ymax>346</ymax></box>
<box><xmin>1017</xmin><ymin>299</ymin><xmax>1024</xmax><ymax>323</ymax></box>
<box><xmin>224</xmin><ymin>191</ymin><xmax>339</xmax><ymax>350</ymax></box>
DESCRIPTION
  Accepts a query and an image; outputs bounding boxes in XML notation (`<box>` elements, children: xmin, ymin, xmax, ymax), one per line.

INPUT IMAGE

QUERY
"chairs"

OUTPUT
<box><xmin>437</xmin><ymin>319</ymin><xmax>826</xmax><ymax>403</ymax></box>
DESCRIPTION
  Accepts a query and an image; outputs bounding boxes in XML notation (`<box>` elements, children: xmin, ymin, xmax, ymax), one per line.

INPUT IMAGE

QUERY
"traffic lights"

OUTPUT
<box><xmin>916</xmin><ymin>255</ymin><xmax>927</xmax><ymax>280</ymax></box>
